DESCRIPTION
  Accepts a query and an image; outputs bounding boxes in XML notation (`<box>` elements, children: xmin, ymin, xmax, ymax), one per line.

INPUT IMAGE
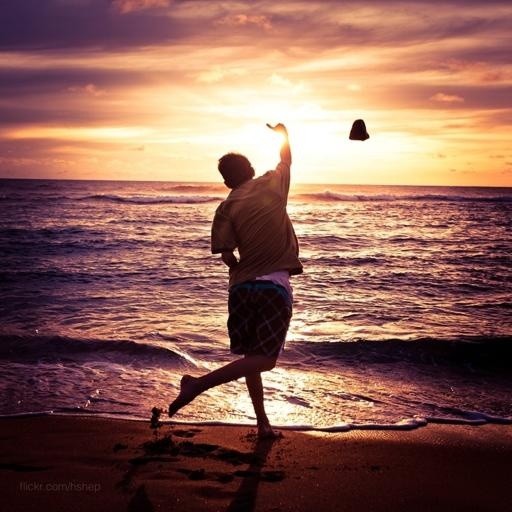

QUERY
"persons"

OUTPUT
<box><xmin>168</xmin><ymin>123</ymin><xmax>304</xmax><ymax>436</ymax></box>
